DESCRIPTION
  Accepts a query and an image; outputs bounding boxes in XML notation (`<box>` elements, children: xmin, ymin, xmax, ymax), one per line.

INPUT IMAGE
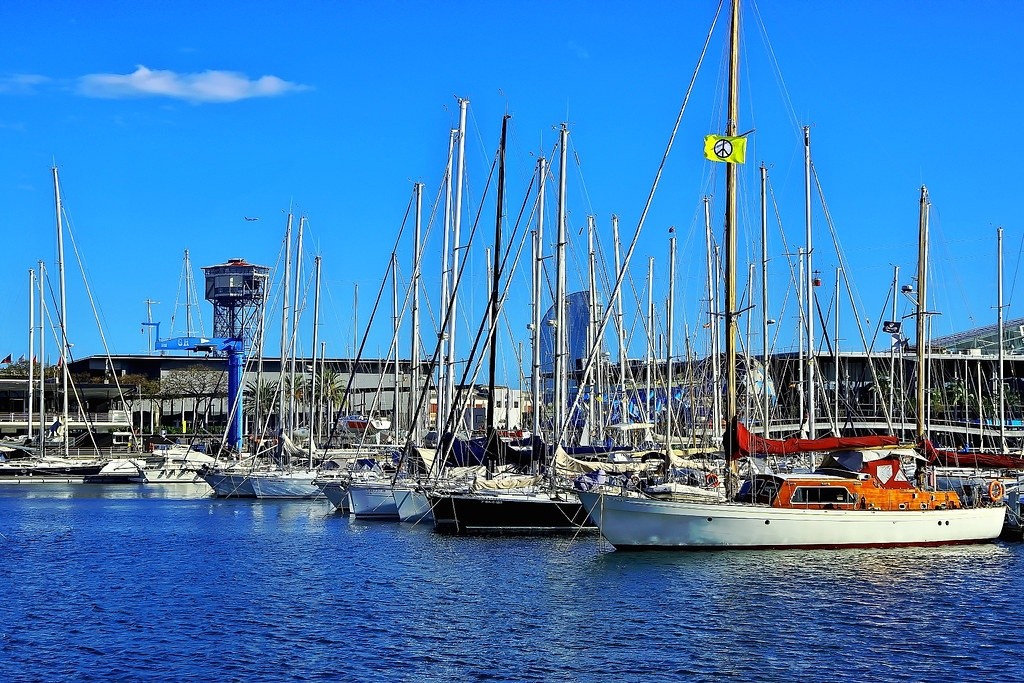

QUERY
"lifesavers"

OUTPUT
<box><xmin>706</xmin><ymin>474</ymin><xmax>719</xmax><ymax>487</ymax></box>
<box><xmin>631</xmin><ymin>475</ymin><xmax>640</xmax><ymax>484</ymax></box>
<box><xmin>990</xmin><ymin>481</ymin><xmax>1002</xmax><ymax>502</ymax></box>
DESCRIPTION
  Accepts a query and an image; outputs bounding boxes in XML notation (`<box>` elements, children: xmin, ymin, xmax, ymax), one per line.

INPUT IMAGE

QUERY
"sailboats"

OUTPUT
<box><xmin>0</xmin><ymin>0</ymin><xmax>1023</xmax><ymax>553</ymax></box>
